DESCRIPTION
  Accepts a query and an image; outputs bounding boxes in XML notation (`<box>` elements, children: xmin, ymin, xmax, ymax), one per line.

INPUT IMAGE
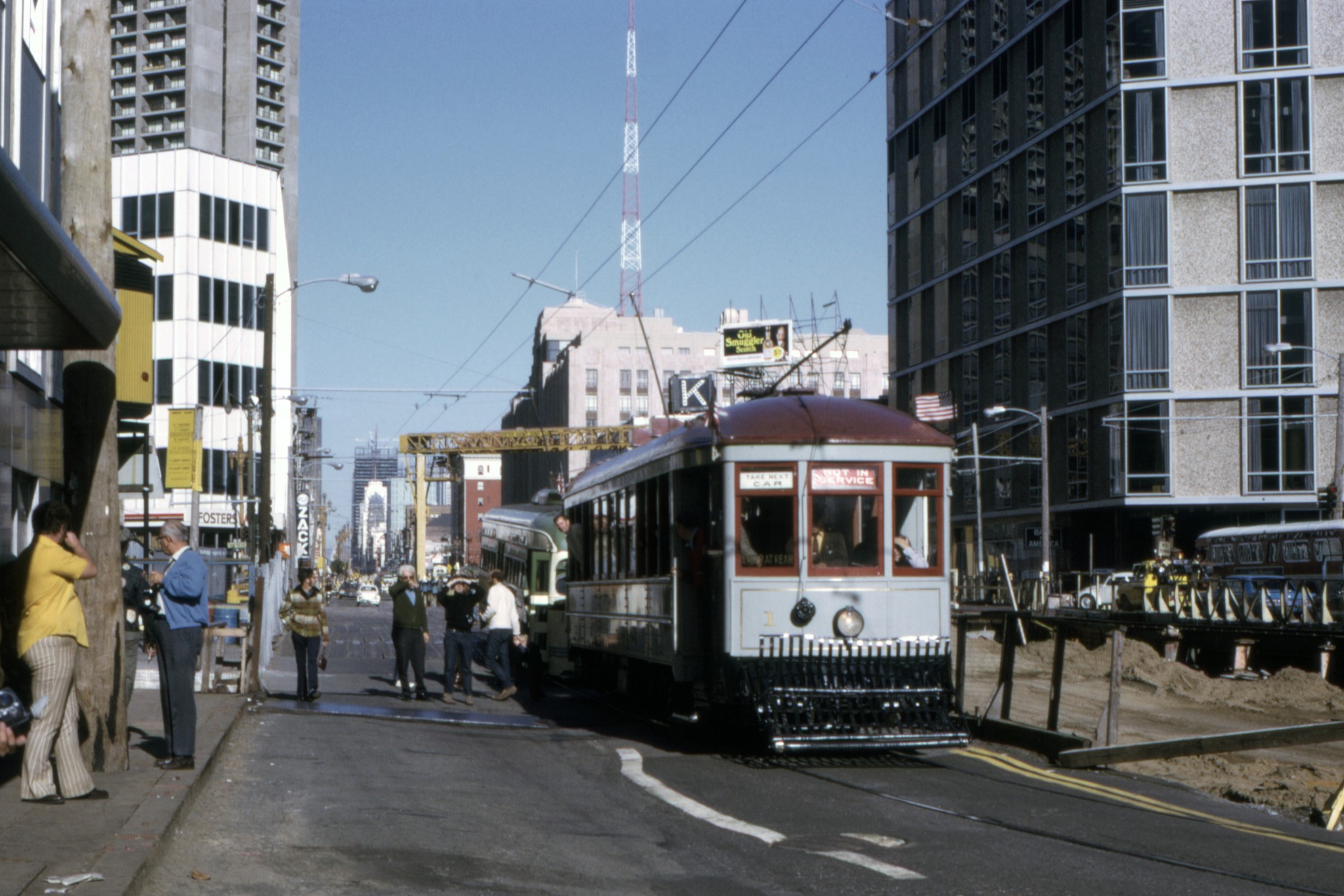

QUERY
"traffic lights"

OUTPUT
<box><xmin>1317</xmin><ymin>487</ymin><xmax>1327</xmax><ymax>510</ymax></box>
<box><xmin>1327</xmin><ymin>484</ymin><xmax>1337</xmax><ymax>510</ymax></box>
<box><xmin>1165</xmin><ymin>517</ymin><xmax>1176</xmax><ymax>538</ymax></box>
<box><xmin>1151</xmin><ymin>517</ymin><xmax>1163</xmax><ymax>538</ymax></box>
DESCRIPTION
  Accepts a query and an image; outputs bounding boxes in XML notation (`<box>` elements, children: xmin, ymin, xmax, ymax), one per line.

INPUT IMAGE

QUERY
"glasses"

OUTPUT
<box><xmin>156</xmin><ymin>535</ymin><xmax>167</xmax><ymax>543</ymax></box>
<box><xmin>399</xmin><ymin>576</ymin><xmax>415</xmax><ymax>580</ymax></box>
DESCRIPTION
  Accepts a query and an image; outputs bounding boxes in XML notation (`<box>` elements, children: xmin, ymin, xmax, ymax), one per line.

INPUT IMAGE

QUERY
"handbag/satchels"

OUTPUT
<box><xmin>318</xmin><ymin>647</ymin><xmax>327</xmax><ymax>670</ymax></box>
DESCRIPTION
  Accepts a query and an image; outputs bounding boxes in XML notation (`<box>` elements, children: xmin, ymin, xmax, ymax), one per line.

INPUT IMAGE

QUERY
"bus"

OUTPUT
<box><xmin>479</xmin><ymin>387</ymin><xmax>576</xmax><ymax>681</ymax></box>
<box><xmin>1195</xmin><ymin>520</ymin><xmax>1344</xmax><ymax>611</ymax></box>
<box><xmin>562</xmin><ymin>294</ymin><xmax>971</xmax><ymax>754</ymax></box>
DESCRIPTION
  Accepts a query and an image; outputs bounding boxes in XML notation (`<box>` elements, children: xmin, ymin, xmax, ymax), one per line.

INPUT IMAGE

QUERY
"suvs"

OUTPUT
<box><xmin>1116</xmin><ymin>557</ymin><xmax>1211</xmax><ymax>612</ymax></box>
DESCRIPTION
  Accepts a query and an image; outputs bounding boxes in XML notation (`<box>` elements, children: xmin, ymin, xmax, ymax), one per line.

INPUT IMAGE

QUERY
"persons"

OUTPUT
<box><xmin>554</xmin><ymin>515</ymin><xmax>590</xmax><ymax>565</ymax></box>
<box><xmin>148</xmin><ymin>521</ymin><xmax>209</xmax><ymax>770</ymax></box>
<box><xmin>671</xmin><ymin>511</ymin><xmax>719</xmax><ymax>680</ymax></box>
<box><xmin>279</xmin><ymin>568</ymin><xmax>329</xmax><ymax>701</ymax></box>
<box><xmin>894</xmin><ymin>535</ymin><xmax>929</xmax><ymax>568</ymax></box>
<box><xmin>740</xmin><ymin>496</ymin><xmax>761</xmax><ymax>566</ymax></box>
<box><xmin>478</xmin><ymin>570</ymin><xmax>521</xmax><ymax>700</ymax></box>
<box><xmin>786</xmin><ymin>502</ymin><xmax>848</xmax><ymax>565</ymax></box>
<box><xmin>850</xmin><ymin>516</ymin><xmax>879</xmax><ymax>565</ymax></box>
<box><xmin>0</xmin><ymin>499</ymin><xmax>109</xmax><ymax>805</ymax></box>
<box><xmin>120</xmin><ymin>525</ymin><xmax>158</xmax><ymax>706</ymax></box>
<box><xmin>389</xmin><ymin>565</ymin><xmax>433</xmax><ymax>702</ymax></box>
<box><xmin>436</xmin><ymin>575</ymin><xmax>486</xmax><ymax>705</ymax></box>
<box><xmin>586</xmin><ymin>515</ymin><xmax>607</xmax><ymax>537</ymax></box>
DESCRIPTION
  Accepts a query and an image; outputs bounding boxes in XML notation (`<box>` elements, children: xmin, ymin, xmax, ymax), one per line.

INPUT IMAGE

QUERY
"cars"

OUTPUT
<box><xmin>338</xmin><ymin>582</ymin><xmax>357</xmax><ymax>600</ymax></box>
<box><xmin>1198</xmin><ymin>574</ymin><xmax>1316</xmax><ymax>620</ymax></box>
<box><xmin>356</xmin><ymin>584</ymin><xmax>381</xmax><ymax>606</ymax></box>
<box><xmin>1075</xmin><ymin>571</ymin><xmax>1143</xmax><ymax>611</ymax></box>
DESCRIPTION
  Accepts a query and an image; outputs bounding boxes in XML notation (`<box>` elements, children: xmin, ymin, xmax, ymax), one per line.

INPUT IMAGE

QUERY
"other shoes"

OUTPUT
<box><xmin>21</xmin><ymin>794</ymin><xmax>64</xmax><ymax>805</ymax></box>
<box><xmin>156</xmin><ymin>755</ymin><xmax>173</xmax><ymax>768</ymax></box>
<box><xmin>409</xmin><ymin>682</ymin><xmax>415</xmax><ymax>688</ymax></box>
<box><xmin>465</xmin><ymin>696</ymin><xmax>473</xmax><ymax>705</ymax></box>
<box><xmin>394</xmin><ymin>679</ymin><xmax>402</xmax><ymax>687</ymax></box>
<box><xmin>298</xmin><ymin>694</ymin><xmax>311</xmax><ymax>702</ymax></box>
<box><xmin>440</xmin><ymin>692</ymin><xmax>455</xmax><ymax>705</ymax></box>
<box><xmin>402</xmin><ymin>690</ymin><xmax>412</xmax><ymax>701</ymax></box>
<box><xmin>310</xmin><ymin>691</ymin><xmax>321</xmax><ymax>699</ymax></box>
<box><xmin>78</xmin><ymin>789</ymin><xmax>108</xmax><ymax>799</ymax></box>
<box><xmin>498</xmin><ymin>685</ymin><xmax>517</xmax><ymax>702</ymax></box>
<box><xmin>417</xmin><ymin>691</ymin><xmax>433</xmax><ymax>702</ymax></box>
<box><xmin>162</xmin><ymin>757</ymin><xmax>195</xmax><ymax>771</ymax></box>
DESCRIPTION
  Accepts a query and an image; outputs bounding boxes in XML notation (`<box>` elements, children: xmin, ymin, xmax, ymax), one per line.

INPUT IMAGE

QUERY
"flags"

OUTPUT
<box><xmin>916</xmin><ymin>391</ymin><xmax>957</xmax><ymax>421</ymax></box>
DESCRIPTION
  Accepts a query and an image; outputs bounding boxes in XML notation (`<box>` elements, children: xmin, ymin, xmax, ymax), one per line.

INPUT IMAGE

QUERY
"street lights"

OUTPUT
<box><xmin>317</xmin><ymin>492</ymin><xmax>354</xmax><ymax>604</ymax></box>
<box><xmin>1262</xmin><ymin>342</ymin><xmax>1344</xmax><ymax>520</ymax></box>
<box><xmin>288</xmin><ymin>448</ymin><xmax>332</xmax><ymax>588</ymax></box>
<box><xmin>983</xmin><ymin>405</ymin><xmax>1052</xmax><ymax>610</ymax></box>
<box><xmin>246</xmin><ymin>396</ymin><xmax>308</xmax><ymax>622</ymax></box>
<box><xmin>249</xmin><ymin>273</ymin><xmax>379</xmax><ymax>694</ymax></box>
